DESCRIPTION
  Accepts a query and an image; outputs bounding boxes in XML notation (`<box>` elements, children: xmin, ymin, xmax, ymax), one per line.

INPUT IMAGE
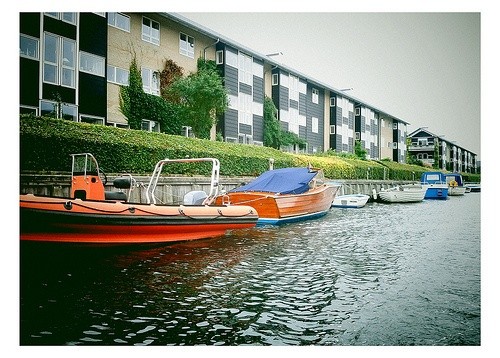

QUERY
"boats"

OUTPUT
<box><xmin>443</xmin><ymin>173</ymin><xmax>480</xmax><ymax>196</ymax></box>
<box><xmin>419</xmin><ymin>171</ymin><xmax>450</xmax><ymax>200</ymax></box>
<box><xmin>378</xmin><ymin>171</ymin><xmax>430</xmax><ymax>203</ymax></box>
<box><xmin>19</xmin><ymin>152</ymin><xmax>260</xmax><ymax>244</ymax></box>
<box><xmin>209</xmin><ymin>157</ymin><xmax>343</xmax><ymax>226</ymax></box>
<box><xmin>330</xmin><ymin>181</ymin><xmax>371</xmax><ymax>208</ymax></box>
